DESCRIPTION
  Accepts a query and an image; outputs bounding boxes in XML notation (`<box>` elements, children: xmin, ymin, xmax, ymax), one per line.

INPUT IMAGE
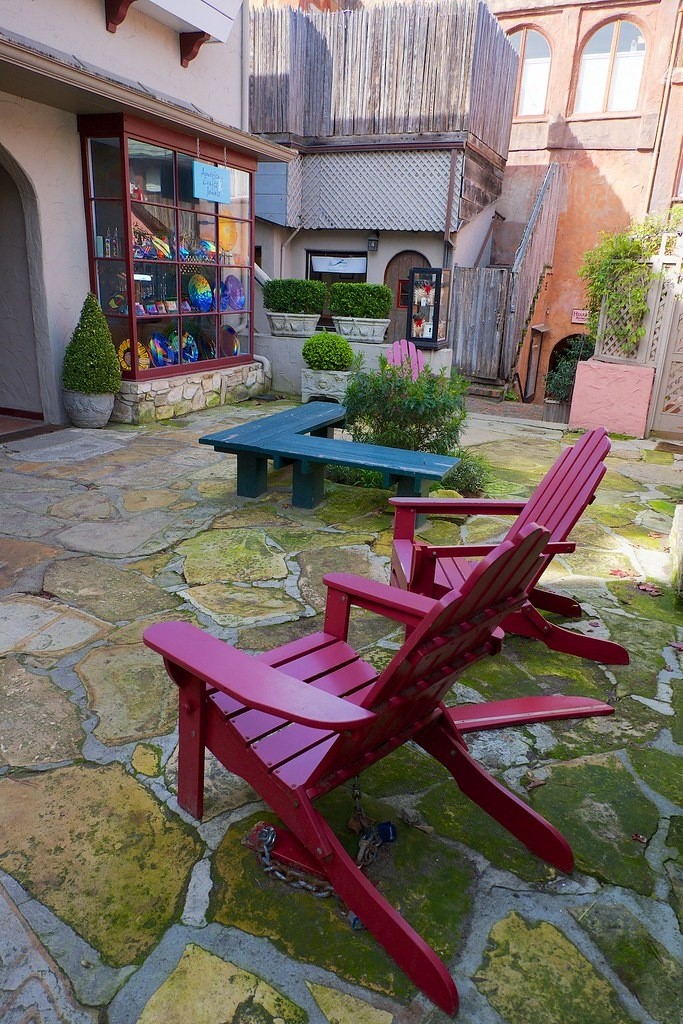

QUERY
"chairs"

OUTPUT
<box><xmin>385</xmin><ymin>429</ymin><xmax>636</xmax><ymax>672</ymax></box>
<box><xmin>141</xmin><ymin>522</ymin><xmax>577</xmax><ymax>1017</ymax></box>
<box><xmin>376</xmin><ymin>339</ymin><xmax>428</xmax><ymax>383</ymax></box>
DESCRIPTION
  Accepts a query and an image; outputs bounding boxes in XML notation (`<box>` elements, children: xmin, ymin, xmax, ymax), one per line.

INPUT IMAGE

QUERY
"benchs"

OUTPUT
<box><xmin>196</xmin><ymin>401</ymin><xmax>461</xmax><ymax>530</ymax></box>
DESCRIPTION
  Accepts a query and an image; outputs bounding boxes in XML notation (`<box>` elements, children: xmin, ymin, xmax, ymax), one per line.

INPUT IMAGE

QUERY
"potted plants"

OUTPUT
<box><xmin>261</xmin><ymin>278</ymin><xmax>328</xmax><ymax>336</ymax></box>
<box><xmin>541</xmin><ymin>332</ymin><xmax>595</xmax><ymax>424</ymax></box>
<box><xmin>327</xmin><ymin>282</ymin><xmax>395</xmax><ymax>345</ymax></box>
<box><xmin>58</xmin><ymin>289</ymin><xmax>124</xmax><ymax>430</ymax></box>
<box><xmin>300</xmin><ymin>332</ymin><xmax>357</xmax><ymax>406</ymax></box>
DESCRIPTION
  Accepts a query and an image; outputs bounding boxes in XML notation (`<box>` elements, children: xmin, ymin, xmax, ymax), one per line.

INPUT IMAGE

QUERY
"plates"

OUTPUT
<box><xmin>118</xmin><ymin>211</ymin><xmax>246</xmax><ymax>372</ymax></box>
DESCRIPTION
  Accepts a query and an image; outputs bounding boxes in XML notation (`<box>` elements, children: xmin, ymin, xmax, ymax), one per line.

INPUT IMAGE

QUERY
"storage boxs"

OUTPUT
<box><xmin>423</xmin><ymin>322</ymin><xmax>433</xmax><ymax>339</ymax></box>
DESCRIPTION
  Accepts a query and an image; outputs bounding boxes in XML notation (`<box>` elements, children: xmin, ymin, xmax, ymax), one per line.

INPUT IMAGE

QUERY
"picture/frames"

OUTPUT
<box><xmin>155</xmin><ymin>301</ymin><xmax>168</xmax><ymax>315</ymax></box>
<box><xmin>165</xmin><ymin>299</ymin><xmax>180</xmax><ymax>314</ymax></box>
<box><xmin>143</xmin><ymin>303</ymin><xmax>159</xmax><ymax>315</ymax></box>
<box><xmin>181</xmin><ymin>297</ymin><xmax>197</xmax><ymax>313</ymax></box>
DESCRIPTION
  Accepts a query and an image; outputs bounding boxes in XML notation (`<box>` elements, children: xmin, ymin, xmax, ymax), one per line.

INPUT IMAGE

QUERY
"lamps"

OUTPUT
<box><xmin>368</xmin><ymin>230</ymin><xmax>379</xmax><ymax>251</ymax></box>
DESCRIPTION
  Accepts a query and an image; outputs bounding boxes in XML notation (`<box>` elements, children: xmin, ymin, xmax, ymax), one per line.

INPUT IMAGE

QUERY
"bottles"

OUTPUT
<box><xmin>105</xmin><ymin>224</ymin><xmax>122</xmax><ymax>258</ymax></box>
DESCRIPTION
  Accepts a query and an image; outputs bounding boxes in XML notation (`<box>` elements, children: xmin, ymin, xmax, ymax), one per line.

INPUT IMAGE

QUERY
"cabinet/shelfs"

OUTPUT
<box><xmin>405</xmin><ymin>267</ymin><xmax>452</xmax><ymax>351</ymax></box>
<box><xmin>76</xmin><ymin>110</ymin><xmax>259</xmax><ymax>382</ymax></box>
